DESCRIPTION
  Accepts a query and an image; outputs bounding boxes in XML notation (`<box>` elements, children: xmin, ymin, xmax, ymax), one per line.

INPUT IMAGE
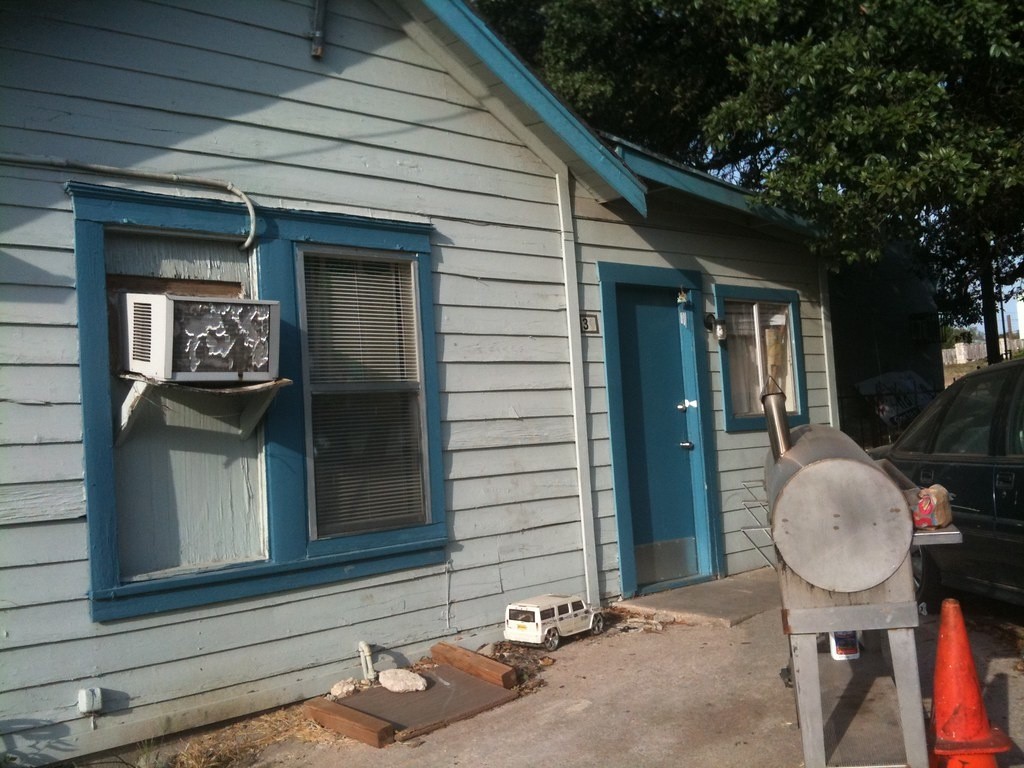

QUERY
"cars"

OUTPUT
<box><xmin>863</xmin><ymin>358</ymin><xmax>1024</xmax><ymax>610</ymax></box>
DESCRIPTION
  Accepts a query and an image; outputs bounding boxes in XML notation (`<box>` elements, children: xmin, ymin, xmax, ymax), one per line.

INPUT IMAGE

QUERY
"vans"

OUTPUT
<box><xmin>503</xmin><ymin>592</ymin><xmax>606</xmax><ymax>653</ymax></box>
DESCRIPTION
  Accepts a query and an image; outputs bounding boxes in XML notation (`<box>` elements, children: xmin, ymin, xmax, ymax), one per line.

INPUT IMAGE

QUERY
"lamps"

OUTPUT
<box><xmin>703</xmin><ymin>312</ymin><xmax>728</xmax><ymax>341</ymax></box>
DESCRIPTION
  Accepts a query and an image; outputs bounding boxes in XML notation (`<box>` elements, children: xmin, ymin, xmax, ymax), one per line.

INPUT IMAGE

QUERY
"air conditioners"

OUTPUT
<box><xmin>119</xmin><ymin>291</ymin><xmax>280</xmax><ymax>384</ymax></box>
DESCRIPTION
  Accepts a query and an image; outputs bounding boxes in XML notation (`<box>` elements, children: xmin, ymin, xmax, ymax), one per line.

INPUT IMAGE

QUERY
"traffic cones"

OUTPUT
<box><xmin>923</xmin><ymin>597</ymin><xmax>1015</xmax><ymax>768</ymax></box>
